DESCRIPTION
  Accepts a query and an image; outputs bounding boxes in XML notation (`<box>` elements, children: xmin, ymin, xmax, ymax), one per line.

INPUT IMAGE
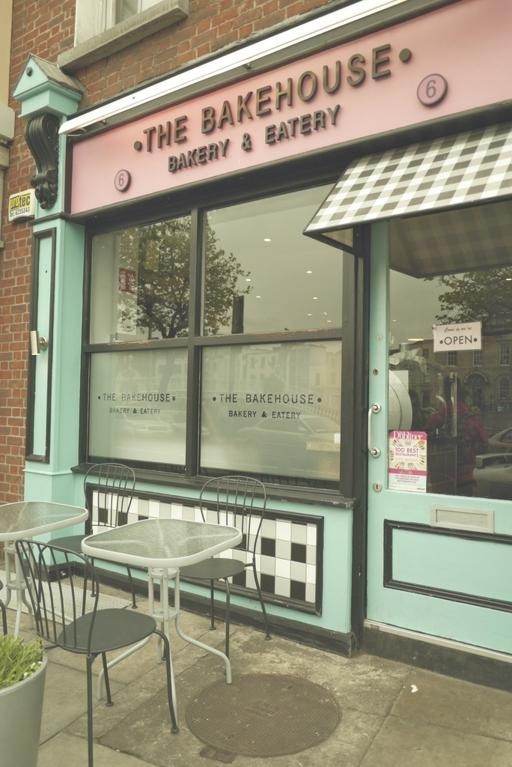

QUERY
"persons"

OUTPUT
<box><xmin>420</xmin><ymin>383</ymin><xmax>490</xmax><ymax>497</ymax></box>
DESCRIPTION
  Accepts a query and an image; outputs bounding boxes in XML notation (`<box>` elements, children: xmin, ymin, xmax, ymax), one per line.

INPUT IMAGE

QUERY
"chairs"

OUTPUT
<box><xmin>197</xmin><ymin>475</ymin><xmax>271</xmax><ymax>678</ymax></box>
<box><xmin>12</xmin><ymin>537</ymin><xmax>177</xmax><ymax>766</ymax></box>
<box><xmin>35</xmin><ymin>464</ymin><xmax>140</xmax><ymax>611</ymax></box>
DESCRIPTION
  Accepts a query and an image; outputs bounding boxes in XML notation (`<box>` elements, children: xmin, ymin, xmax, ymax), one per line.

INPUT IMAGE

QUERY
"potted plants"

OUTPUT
<box><xmin>1</xmin><ymin>626</ymin><xmax>50</xmax><ymax>767</ymax></box>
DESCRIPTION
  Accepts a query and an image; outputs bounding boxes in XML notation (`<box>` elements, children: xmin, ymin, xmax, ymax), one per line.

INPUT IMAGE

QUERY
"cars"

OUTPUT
<box><xmin>108</xmin><ymin>406</ymin><xmax>210</xmax><ymax>445</ymax></box>
<box><xmin>472</xmin><ymin>427</ymin><xmax>512</xmax><ymax>488</ymax></box>
<box><xmin>235</xmin><ymin>413</ymin><xmax>341</xmax><ymax>469</ymax></box>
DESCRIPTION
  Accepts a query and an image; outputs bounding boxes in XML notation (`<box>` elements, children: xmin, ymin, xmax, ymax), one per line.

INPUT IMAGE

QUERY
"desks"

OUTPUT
<box><xmin>0</xmin><ymin>500</ymin><xmax>90</xmax><ymax>643</ymax></box>
<box><xmin>79</xmin><ymin>517</ymin><xmax>246</xmax><ymax>703</ymax></box>
